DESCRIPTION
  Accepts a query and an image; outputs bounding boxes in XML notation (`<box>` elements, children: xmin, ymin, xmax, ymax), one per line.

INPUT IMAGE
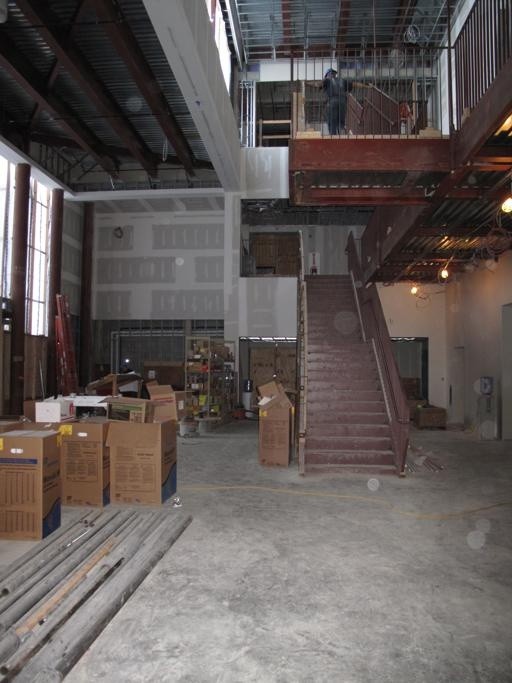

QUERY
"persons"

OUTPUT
<box><xmin>305</xmin><ymin>68</ymin><xmax>370</xmax><ymax>135</ymax></box>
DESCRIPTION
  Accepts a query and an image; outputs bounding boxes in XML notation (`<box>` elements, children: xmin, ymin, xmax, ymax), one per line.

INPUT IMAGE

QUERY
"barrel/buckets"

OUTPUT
<box><xmin>179</xmin><ymin>421</ymin><xmax>200</xmax><ymax>437</ymax></box>
<box><xmin>479</xmin><ymin>375</ymin><xmax>492</xmax><ymax>395</ymax></box>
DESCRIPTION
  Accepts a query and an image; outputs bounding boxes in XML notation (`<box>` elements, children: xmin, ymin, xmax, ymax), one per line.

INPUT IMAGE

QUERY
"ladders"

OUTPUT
<box><xmin>54</xmin><ymin>293</ymin><xmax>77</xmax><ymax>397</ymax></box>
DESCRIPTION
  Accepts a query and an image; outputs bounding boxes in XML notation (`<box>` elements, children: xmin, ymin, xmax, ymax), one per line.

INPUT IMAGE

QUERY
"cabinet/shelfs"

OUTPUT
<box><xmin>184</xmin><ymin>333</ymin><xmax>240</xmax><ymax>427</ymax></box>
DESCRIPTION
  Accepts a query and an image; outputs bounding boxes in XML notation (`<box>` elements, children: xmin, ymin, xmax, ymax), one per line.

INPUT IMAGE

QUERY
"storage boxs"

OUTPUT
<box><xmin>254</xmin><ymin>380</ymin><xmax>294</xmax><ymax>469</ymax></box>
<box><xmin>1</xmin><ymin>373</ymin><xmax>196</xmax><ymax>545</ymax></box>
